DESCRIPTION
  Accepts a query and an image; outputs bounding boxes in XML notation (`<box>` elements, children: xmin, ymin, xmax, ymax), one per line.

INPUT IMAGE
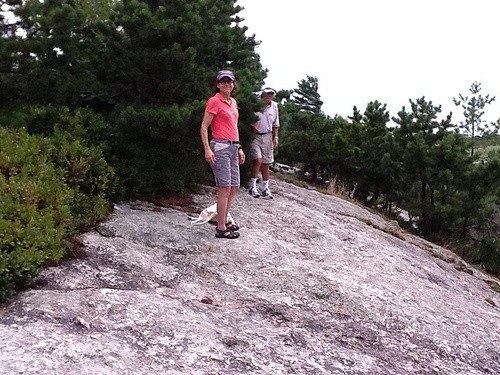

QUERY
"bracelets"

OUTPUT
<box><xmin>239</xmin><ymin>147</ymin><xmax>242</xmax><ymax>149</ymax></box>
<box><xmin>274</xmin><ymin>136</ymin><xmax>278</xmax><ymax>138</ymax></box>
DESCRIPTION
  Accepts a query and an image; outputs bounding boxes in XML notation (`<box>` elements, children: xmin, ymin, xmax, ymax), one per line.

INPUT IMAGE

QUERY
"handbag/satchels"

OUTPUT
<box><xmin>191</xmin><ymin>203</ymin><xmax>235</xmax><ymax>226</ymax></box>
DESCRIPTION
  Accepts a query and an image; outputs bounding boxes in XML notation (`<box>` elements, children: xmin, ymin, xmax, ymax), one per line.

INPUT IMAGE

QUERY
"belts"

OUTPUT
<box><xmin>228</xmin><ymin>141</ymin><xmax>239</xmax><ymax>144</ymax></box>
<box><xmin>257</xmin><ymin>133</ymin><xmax>266</xmax><ymax>135</ymax></box>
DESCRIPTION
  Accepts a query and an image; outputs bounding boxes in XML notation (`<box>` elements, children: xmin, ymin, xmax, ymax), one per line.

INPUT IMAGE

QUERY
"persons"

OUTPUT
<box><xmin>201</xmin><ymin>70</ymin><xmax>245</xmax><ymax>239</ymax></box>
<box><xmin>248</xmin><ymin>87</ymin><xmax>281</xmax><ymax>200</ymax></box>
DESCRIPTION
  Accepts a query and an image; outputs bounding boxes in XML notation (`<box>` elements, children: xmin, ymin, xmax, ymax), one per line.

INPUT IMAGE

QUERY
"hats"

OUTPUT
<box><xmin>264</xmin><ymin>87</ymin><xmax>276</xmax><ymax>95</ymax></box>
<box><xmin>217</xmin><ymin>70</ymin><xmax>235</xmax><ymax>80</ymax></box>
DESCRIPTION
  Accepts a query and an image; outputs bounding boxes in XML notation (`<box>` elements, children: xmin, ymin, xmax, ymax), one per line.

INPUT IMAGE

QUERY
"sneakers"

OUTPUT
<box><xmin>249</xmin><ymin>189</ymin><xmax>260</xmax><ymax>198</ymax></box>
<box><xmin>262</xmin><ymin>189</ymin><xmax>273</xmax><ymax>199</ymax></box>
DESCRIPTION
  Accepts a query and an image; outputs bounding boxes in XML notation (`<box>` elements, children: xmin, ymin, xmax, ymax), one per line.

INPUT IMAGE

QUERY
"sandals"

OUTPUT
<box><xmin>215</xmin><ymin>227</ymin><xmax>240</xmax><ymax>238</ymax></box>
<box><xmin>225</xmin><ymin>222</ymin><xmax>239</xmax><ymax>231</ymax></box>
